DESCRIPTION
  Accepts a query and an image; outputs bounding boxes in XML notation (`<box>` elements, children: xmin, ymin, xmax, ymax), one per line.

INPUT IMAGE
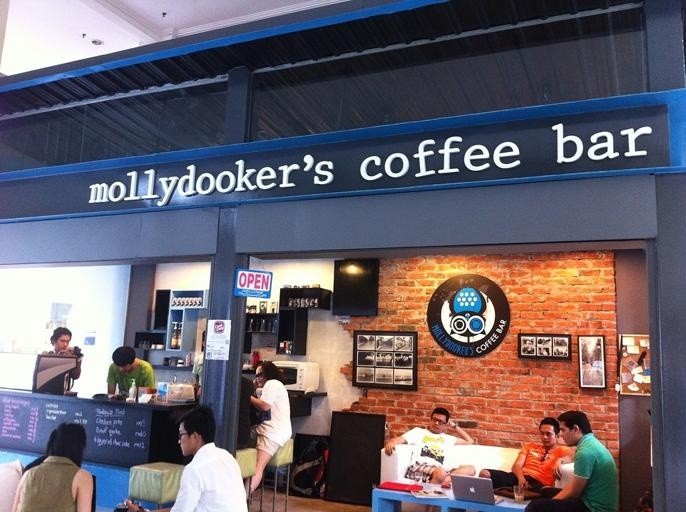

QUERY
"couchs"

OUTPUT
<box><xmin>380</xmin><ymin>444</ymin><xmax>575</xmax><ymax>490</ymax></box>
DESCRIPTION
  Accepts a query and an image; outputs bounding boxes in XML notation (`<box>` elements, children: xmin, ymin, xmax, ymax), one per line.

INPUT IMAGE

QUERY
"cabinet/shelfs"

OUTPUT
<box><xmin>130</xmin><ymin>288</ymin><xmax>330</xmax><ymax>417</ymax></box>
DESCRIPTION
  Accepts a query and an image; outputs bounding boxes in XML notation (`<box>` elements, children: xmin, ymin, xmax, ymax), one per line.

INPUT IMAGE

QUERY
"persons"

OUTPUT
<box><xmin>384</xmin><ymin>408</ymin><xmax>476</xmax><ymax>489</ymax></box>
<box><xmin>479</xmin><ymin>417</ymin><xmax>575</xmax><ymax>493</ymax></box>
<box><xmin>124</xmin><ymin>407</ymin><xmax>249</xmax><ymax>512</ymax></box>
<box><xmin>245</xmin><ymin>360</ymin><xmax>292</xmax><ymax>498</ymax></box>
<box><xmin>192</xmin><ymin>331</ymin><xmax>206</xmax><ymax>400</ymax></box>
<box><xmin>12</xmin><ymin>423</ymin><xmax>94</xmax><ymax>512</ymax></box>
<box><xmin>637</xmin><ymin>351</ymin><xmax>647</xmax><ymax>370</ymax></box>
<box><xmin>525</xmin><ymin>409</ymin><xmax>620</xmax><ymax>512</ymax></box>
<box><xmin>42</xmin><ymin>327</ymin><xmax>82</xmax><ymax>395</ymax></box>
<box><xmin>236</xmin><ymin>375</ymin><xmax>254</xmax><ymax>449</ymax></box>
<box><xmin>106</xmin><ymin>347</ymin><xmax>155</xmax><ymax>400</ymax></box>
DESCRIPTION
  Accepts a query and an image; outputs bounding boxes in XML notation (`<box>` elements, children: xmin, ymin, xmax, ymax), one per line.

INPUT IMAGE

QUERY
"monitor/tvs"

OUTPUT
<box><xmin>332</xmin><ymin>259</ymin><xmax>379</xmax><ymax>316</ymax></box>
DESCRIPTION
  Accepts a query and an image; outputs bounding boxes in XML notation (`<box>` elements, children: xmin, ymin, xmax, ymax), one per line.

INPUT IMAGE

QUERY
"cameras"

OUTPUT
<box><xmin>72</xmin><ymin>346</ymin><xmax>84</xmax><ymax>358</ymax></box>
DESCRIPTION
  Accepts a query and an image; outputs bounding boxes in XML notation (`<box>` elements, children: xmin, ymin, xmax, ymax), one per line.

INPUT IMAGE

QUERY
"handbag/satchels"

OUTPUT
<box><xmin>265</xmin><ymin>457</ymin><xmax>294</xmax><ymax>490</ymax></box>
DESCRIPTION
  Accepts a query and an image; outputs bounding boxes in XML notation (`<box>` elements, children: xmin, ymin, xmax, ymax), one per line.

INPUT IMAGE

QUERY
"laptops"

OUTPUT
<box><xmin>450</xmin><ymin>473</ymin><xmax>504</xmax><ymax>506</ymax></box>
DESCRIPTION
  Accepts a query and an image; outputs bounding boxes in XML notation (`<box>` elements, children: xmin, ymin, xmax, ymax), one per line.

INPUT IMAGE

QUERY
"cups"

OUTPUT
<box><xmin>512</xmin><ymin>485</ymin><xmax>525</xmax><ymax>503</ymax></box>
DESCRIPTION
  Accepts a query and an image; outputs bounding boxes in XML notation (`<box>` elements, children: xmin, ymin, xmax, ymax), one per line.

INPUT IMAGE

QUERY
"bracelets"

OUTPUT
<box><xmin>452</xmin><ymin>422</ymin><xmax>458</xmax><ymax>429</ymax></box>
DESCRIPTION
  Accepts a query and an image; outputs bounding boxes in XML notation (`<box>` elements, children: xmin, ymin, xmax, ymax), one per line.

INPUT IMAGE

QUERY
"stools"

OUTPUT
<box><xmin>236</xmin><ymin>447</ymin><xmax>257</xmax><ymax>512</ymax></box>
<box><xmin>127</xmin><ymin>462</ymin><xmax>185</xmax><ymax>510</ymax></box>
<box><xmin>264</xmin><ymin>438</ymin><xmax>294</xmax><ymax>511</ymax></box>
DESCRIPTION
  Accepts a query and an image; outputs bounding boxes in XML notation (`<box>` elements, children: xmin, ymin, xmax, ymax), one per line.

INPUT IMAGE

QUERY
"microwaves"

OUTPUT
<box><xmin>273</xmin><ymin>360</ymin><xmax>319</xmax><ymax>395</ymax></box>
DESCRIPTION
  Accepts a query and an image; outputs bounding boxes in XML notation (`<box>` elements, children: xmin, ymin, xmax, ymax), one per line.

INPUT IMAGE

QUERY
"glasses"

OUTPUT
<box><xmin>254</xmin><ymin>372</ymin><xmax>263</xmax><ymax>378</ymax></box>
<box><xmin>177</xmin><ymin>433</ymin><xmax>187</xmax><ymax>439</ymax></box>
<box><xmin>541</xmin><ymin>449</ymin><xmax>548</xmax><ymax>462</ymax></box>
<box><xmin>431</xmin><ymin>416</ymin><xmax>446</xmax><ymax>424</ymax></box>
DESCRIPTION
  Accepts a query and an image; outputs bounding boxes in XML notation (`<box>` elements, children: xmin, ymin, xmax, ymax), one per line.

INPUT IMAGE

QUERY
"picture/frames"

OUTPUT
<box><xmin>577</xmin><ymin>335</ymin><xmax>606</xmax><ymax>389</ymax></box>
<box><xmin>518</xmin><ymin>333</ymin><xmax>571</xmax><ymax>361</ymax></box>
<box><xmin>618</xmin><ymin>334</ymin><xmax>652</xmax><ymax>397</ymax></box>
<box><xmin>352</xmin><ymin>330</ymin><xmax>417</xmax><ymax>391</ymax></box>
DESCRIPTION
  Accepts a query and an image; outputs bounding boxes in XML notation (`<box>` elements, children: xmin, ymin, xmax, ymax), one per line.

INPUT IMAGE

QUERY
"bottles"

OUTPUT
<box><xmin>171</xmin><ymin>321</ymin><xmax>181</xmax><ymax>349</ymax></box>
<box><xmin>252</xmin><ymin>351</ymin><xmax>260</xmax><ymax>368</ymax></box>
<box><xmin>260</xmin><ymin>301</ymin><xmax>278</xmax><ymax>313</ymax></box>
<box><xmin>128</xmin><ymin>378</ymin><xmax>137</xmax><ymax>398</ymax></box>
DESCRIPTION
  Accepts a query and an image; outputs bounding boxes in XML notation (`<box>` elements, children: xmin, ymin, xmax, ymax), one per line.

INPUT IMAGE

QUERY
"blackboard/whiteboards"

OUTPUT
<box><xmin>0</xmin><ymin>391</ymin><xmax>153</xmax><ymax>468</ymax></box>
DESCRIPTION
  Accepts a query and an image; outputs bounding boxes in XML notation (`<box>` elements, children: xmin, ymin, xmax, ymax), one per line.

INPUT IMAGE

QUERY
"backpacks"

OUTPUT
<box><xmin>289</xmin><ymin>437</ymin><xmax>330</xmax><ymax>499</ymax></box>
